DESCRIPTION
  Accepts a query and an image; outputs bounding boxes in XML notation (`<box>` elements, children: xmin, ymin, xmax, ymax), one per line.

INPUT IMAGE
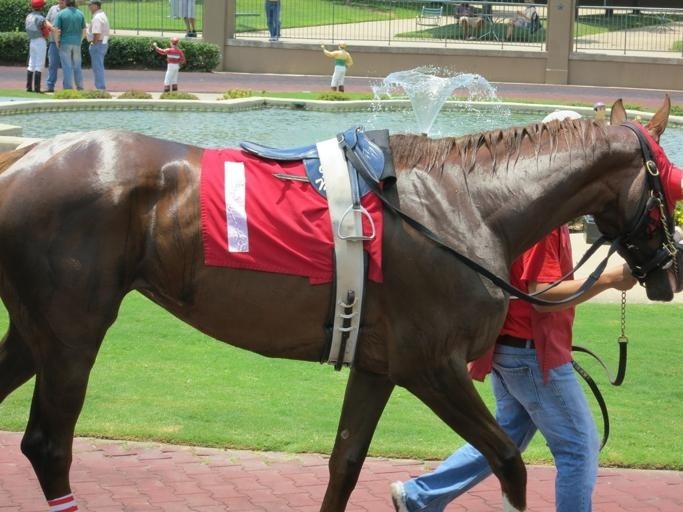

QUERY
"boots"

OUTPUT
<box><xmin>35</xmin><ymin>71</ymin><xmax>41</xmax><ymax>92</ymax></box>
<box><xmin>26</xmin><ymin>71</ymin><xmax>32</xmax><ymax>91</ymax></box>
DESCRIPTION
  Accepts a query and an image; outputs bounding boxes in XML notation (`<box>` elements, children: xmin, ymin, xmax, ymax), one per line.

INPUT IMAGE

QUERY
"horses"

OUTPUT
<box><xmin>0</xmin><ymin>93</ymin><xmax>683</xmax><ymax>512</ymax></box>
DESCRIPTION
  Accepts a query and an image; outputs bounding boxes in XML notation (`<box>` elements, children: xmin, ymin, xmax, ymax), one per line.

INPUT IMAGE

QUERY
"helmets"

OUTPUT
<box><xmin>32</xmin><ymin>0</ymin><xmax>44</xmax><ymax>8</ymax></box>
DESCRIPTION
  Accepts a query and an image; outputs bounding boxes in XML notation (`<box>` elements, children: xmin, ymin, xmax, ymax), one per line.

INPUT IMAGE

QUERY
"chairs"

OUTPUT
<box><xmin>415</xmin><ymin>5</ymin><xmax>444</xmax><ymax>40</ymax></box>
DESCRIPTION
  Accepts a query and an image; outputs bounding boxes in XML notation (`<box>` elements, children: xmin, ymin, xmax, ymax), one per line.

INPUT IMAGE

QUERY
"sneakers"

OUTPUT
<box><xmin>391</xmin><ymin>481</ymin><xmax>409</xmax><ymax>512</ymax></box>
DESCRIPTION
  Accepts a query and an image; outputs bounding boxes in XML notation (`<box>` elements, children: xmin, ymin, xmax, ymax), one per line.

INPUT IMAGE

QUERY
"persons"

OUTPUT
<box><xmin>24</xmin><ymin>0</ymin><xmax>60</xmax><ymax>93</ymax></box>
<box><xmin>53</xmin><ymin>0</ymin><xmax>86</xmax><ymax>93</ymax></box>
<box><xmin>169</xmin><ymin>0</ymin><xmax>198</xmax><ymax>38</ymax></box>
<box><xmin>85</xmin><ymin>0</ymin><xmax>110</xmax><ymax>91</ymax></box>
<box><xmin>390</xmin><ymin>111</ymin><xmax>634</xmax><ymax>512</ymax></box>
<box><xmin>151</xmin><ymin>38</ymin><xmax>186</xmax><ymax>93</ymax></box>
<box><xmin>505</xmin><ymin>0</ymin><xmax>537</xmax><ymax>41</ymax></box>
<box><xmin>455</xmin><ymin>0</ymin><xmax>483</xmax><ymax>41</ymax></box>
<box><xmin>321</xmin><ymin>42</ymin><xmax>352</xmax><ymax>92</ymax></box>
<box><xmin>46</xmin><ymin>0</ymin><xmax>64</xmax><ymax>93</ymax></box>
<box><xmin>264</xmin><ymin>0</ymin><xmax>281</xmax><ymax>42</ymax></box>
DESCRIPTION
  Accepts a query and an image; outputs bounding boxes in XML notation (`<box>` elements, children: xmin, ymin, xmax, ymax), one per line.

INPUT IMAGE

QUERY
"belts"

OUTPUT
<box><xmin>498</xmin><ymin>335</ymin><xmax>535</xmax><ymax>348</ymax></box>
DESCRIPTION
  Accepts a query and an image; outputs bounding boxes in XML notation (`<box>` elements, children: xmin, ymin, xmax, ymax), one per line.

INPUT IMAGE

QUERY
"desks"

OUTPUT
<box><xmin>474</xmin><ymin>13</ymin><xmax>517</xmax><ymax>42</ymax></box>
